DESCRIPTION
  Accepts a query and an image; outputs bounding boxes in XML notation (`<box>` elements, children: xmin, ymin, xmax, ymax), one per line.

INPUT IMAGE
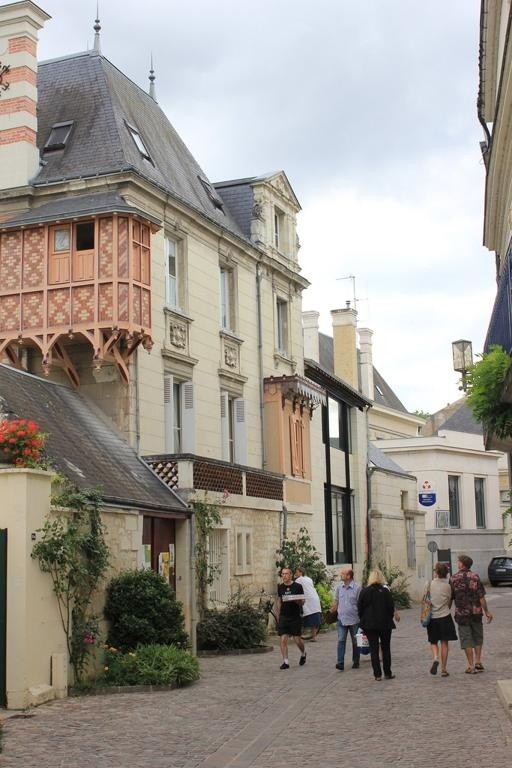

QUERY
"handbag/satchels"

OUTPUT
<box><xmin>355</xmin><ymin>624</ymin><xmax>372</xmax><ymax>655</ymax></box>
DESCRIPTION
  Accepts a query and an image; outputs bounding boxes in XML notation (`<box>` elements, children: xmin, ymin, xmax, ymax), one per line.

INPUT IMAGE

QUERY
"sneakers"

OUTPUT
<box><xmin>299</xmin><ymin>652</ymin><xmax>307</xmax><ymax>666</ymax></box>
<box><xmin>384</xmin><ymin>674</ymin><xmax>396</xmax><ymax>680</ymax></box>
<box><xmin>464</xmin><ymin>662</ymin><xmax>484</xmax><ymax>674</ymax></box>
<box><xmin>375</xmin><ymin>676</ymin><xmax>382</xmax><ymax>681</ymax></box>
<box><xmin>309</xmin><ymin>638</ymin><xmax>318</xmax><ymax>642</ymax></box>
<box><xmin>419</xmin><ymin>580</ymin><xmax>433</xmax><ymax>628</ymax></box>
<box><xmin>440</xmin><ymin>668</ymin><xmax>449</xmax><ymax>678</ymax></box>
<box><xmin>429</xmin><ymin>656</ymin><xmax>439</xmax><ymax>676</ymax></box>
<box><xmin>351</xmin><ymin>662</ymin><xmax>360</xmax><ymax>669</ymax></box>
<box><xmin>335</xmin><ymin>662</ymin><xmax>345</xmax><ymax>670</ymax></box>
<box><xmin>280</xmin><ymin>662</ymin><xmax>289</xmax><ymax>670</ymax></box>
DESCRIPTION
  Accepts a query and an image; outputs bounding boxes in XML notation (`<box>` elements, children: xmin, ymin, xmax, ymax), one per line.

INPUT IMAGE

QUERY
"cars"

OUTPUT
<box><xmin>487</xmin><ymin>556</ymin><xmax>511</xmax><ymax>586</ymax></box>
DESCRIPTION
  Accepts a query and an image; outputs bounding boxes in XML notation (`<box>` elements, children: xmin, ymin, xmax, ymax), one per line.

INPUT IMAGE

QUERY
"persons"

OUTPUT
<box><xmin>276</xmin><ymin>567</ymin><xmax>307</xmax><ymax>669</ymax></box>
<box><xmin>449</xmin><ymin>554</ymin><xmax>493</xmax><ymax>674</ymax></box>
<box><xmin>294</xmin><ymin>567</ymin><xmax>322</xmax><ymax>642</ymax></box>
<box><xmin>422</xmin><ymin>562</ymin><xmax>453</xmax><ymax>677</ymax></box>
<box><xmin>357</xmin><ymin>568</ymin><xmax>401</xmax><ymax>681</ymax></box>
<box><xmin>329</xmin><ymin>567</ymin><xmax>363</xmax><ymax>670</ymax></box>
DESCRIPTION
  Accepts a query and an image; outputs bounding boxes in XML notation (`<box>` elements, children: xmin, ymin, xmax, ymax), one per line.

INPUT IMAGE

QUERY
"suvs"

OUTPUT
<box><xmin>257</xmin><ymin>588</ymin><xmax>320</xmax><ymax>640</ymax></box>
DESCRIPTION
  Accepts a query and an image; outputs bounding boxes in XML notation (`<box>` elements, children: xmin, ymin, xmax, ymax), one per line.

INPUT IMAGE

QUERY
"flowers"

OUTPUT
<box><xmin>1</xmin><ymin>414</ymin><xmax>54</xmax><ymax>472</ymax></box>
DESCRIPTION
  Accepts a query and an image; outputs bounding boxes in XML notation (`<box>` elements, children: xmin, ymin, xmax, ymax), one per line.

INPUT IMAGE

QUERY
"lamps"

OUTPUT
<box><xmin>450</xmin><ymin>339</ymin><xmax>475</xmax><ymax>392</ymax></box>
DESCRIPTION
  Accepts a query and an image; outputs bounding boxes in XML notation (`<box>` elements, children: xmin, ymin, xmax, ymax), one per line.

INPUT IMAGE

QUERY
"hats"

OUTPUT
<box><xmin>456</xmin><ymin>554</ymin><xmax>474</xmax><ymax>568</ymax></box>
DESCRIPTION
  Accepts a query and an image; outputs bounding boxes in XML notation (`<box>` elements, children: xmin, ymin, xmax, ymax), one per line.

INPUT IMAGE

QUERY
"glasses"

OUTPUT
<box><xmin>281</xmin><ymin>573</ymin><xmax>291</xmax><ymax>576</ymax></box>
